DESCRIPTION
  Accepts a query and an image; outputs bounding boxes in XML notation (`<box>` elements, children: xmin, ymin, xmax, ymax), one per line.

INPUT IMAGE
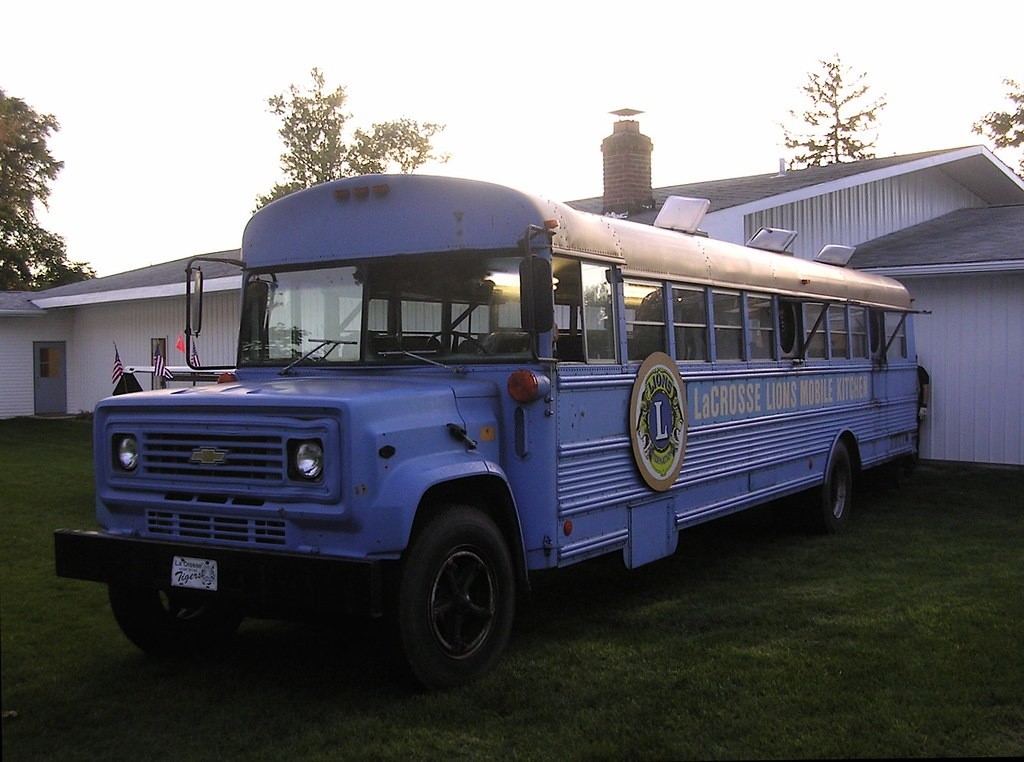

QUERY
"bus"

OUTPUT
<box><xmin>54</xmin><ymin>172</ymin><xmax>934</xmax><ymax>677</ymax></box>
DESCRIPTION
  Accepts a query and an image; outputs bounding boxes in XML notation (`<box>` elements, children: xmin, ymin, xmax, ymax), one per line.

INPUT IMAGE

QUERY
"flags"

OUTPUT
<box><xmin>112</xmin><ymin>344</ymin><xmax>124</xmax><ymax>384</ymax></box>
<box><xmin>175</xmin><ymin>328</ymin><xmax>201</xmax><ymax>376</ymax></box>
<box><xmin>153</xmin><ymin>343</ymin><xmax>174</xmax><ymax>380</ymax></box>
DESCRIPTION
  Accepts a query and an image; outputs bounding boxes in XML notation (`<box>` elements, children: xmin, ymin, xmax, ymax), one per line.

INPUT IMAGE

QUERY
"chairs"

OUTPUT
<box><xmin>478</xmin><ymin>331</ymin><xmax>532</xmax><ymax>353</ymax></box>
